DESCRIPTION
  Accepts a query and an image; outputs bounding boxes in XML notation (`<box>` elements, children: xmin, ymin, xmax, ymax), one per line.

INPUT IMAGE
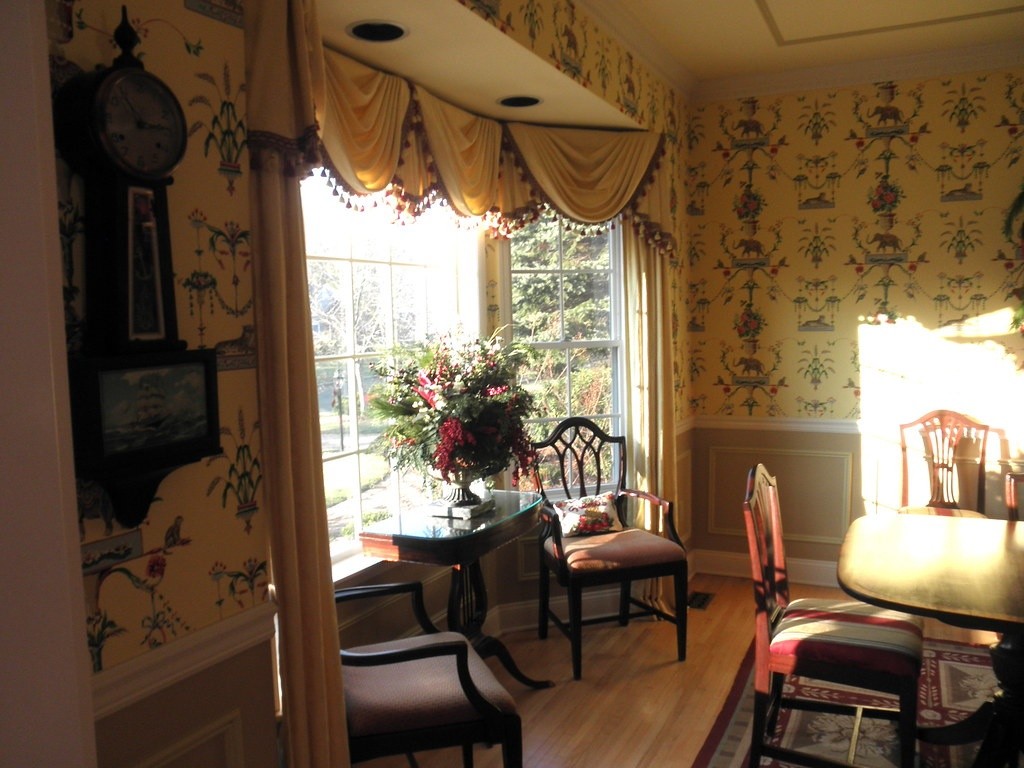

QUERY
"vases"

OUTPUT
<box><xmin>425</xmin><ymin>463</ymin><xmax>495</xmax><ymax>520</ymax></box>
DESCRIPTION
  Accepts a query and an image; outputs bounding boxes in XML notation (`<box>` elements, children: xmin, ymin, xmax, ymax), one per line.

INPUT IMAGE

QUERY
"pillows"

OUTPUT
<box><xmin>553</xmin><ymin>491</ymin><xmax>623</xmax><ymax>538</ymax></box>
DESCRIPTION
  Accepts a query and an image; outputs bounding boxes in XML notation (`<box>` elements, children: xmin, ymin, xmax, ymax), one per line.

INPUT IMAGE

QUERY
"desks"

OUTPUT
<box><xmin>836</xmin><ymin>513</ymin><xmax>1024</xmax><ymax>768</ymax></box>
<box><xmin>358</xmin><ymin>491</ymin><xmax>556</xmax><ymax>687</ymax></box>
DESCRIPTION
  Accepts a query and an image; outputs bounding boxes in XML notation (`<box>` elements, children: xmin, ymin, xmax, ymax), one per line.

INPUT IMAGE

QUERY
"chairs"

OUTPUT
<box><xmin>741</xmin><ymin>463</ymin><xmax>923</xmax><ymax>768</ymax></box>
<box><xmin>525</xmin><ymin>417</ymin><xmax>688</xmax><ymax>681</ymax></box>
<box><xmin>332</xmin><ymin>580</ymin><xmax>521</xmax><ymax>768</ymax></box>
<box><xmin>899</xmin><ymin>411</ymin><xmax>989</xmax><ymax>518</ymax></box>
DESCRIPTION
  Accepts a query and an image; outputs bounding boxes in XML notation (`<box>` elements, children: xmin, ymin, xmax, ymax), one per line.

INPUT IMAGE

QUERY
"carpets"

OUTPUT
<box><xmin>691</xmin><ymin>634</ymin><xmax>1024</xmax><ymax>768</ymax></box>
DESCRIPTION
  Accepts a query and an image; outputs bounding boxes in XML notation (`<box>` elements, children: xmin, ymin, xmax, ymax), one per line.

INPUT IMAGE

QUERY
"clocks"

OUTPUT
<box><xmin>66</xmin><ymin>3</ymin><xmax>225</xmax><ymax>530</ymax></box>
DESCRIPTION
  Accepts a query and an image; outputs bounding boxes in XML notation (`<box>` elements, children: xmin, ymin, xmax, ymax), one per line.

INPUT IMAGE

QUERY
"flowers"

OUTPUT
<box><xmin>366</xmin><ymin>324</ymin><xmax>541</xmax><ymax>487</ymax></box>
